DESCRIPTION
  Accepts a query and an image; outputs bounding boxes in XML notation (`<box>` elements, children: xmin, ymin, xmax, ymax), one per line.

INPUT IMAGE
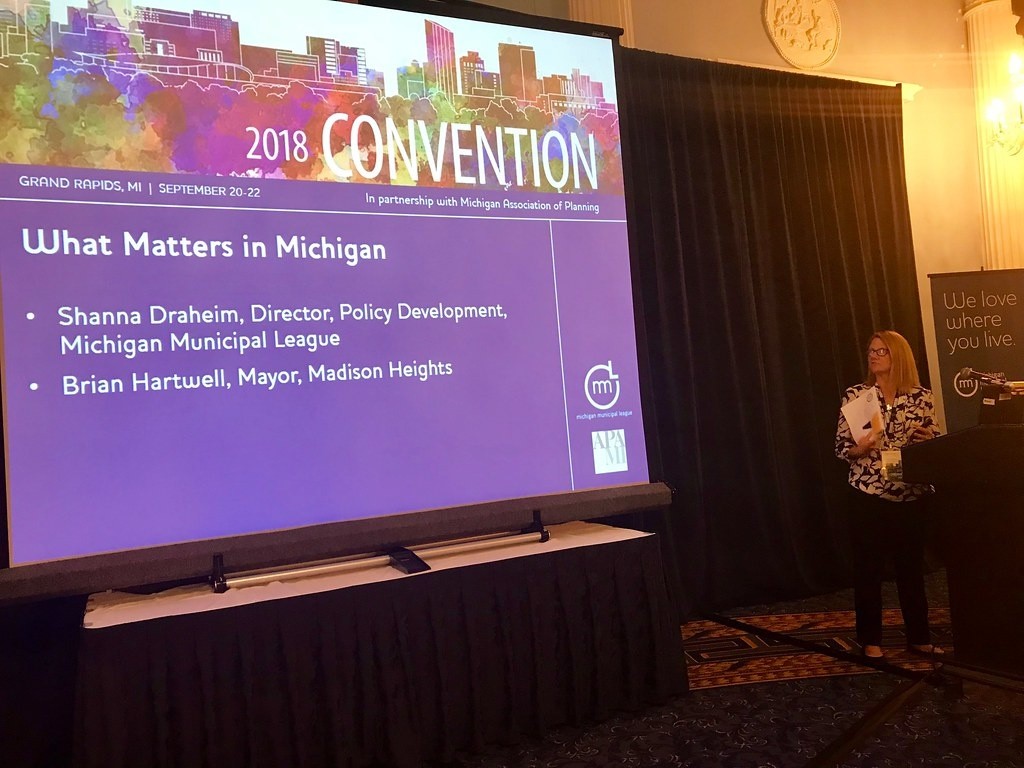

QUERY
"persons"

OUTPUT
<box><xmin>835</xmin><ymin>331</ymin><xmax>946</xmax><ymax>668</ymax></box>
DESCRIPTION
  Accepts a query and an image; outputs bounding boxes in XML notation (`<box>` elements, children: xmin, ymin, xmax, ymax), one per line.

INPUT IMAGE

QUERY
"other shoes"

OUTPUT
<box><xmin>863</xmin><ymin>643</ymin><xmax>883</xmax><ymax>658</ymax></box>
<box><xmin>912</xmin><ymin>644</ymin><xmax>944</xmax><ymax>654</ymax></box>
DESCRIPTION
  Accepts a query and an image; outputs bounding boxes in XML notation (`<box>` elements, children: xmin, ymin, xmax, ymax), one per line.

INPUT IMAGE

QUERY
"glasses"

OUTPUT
<box><xmin>866</xmin><ymin>348</ymin><xmax>889</xmax><ymax>357</ymax></box>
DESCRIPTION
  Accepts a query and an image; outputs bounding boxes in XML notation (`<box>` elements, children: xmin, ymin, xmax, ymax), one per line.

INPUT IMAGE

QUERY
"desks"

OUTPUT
<box><xmin>71</xmin><ymin>521</ymin><xmax>691</xmax><ymax>768</ymax></box>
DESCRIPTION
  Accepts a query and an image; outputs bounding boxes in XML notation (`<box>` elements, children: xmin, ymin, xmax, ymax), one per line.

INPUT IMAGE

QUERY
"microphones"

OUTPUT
<box><xmin>960</xmin><ymin>367</ymin><xmax>1014</xmax><ymax>388</ymax></box>
